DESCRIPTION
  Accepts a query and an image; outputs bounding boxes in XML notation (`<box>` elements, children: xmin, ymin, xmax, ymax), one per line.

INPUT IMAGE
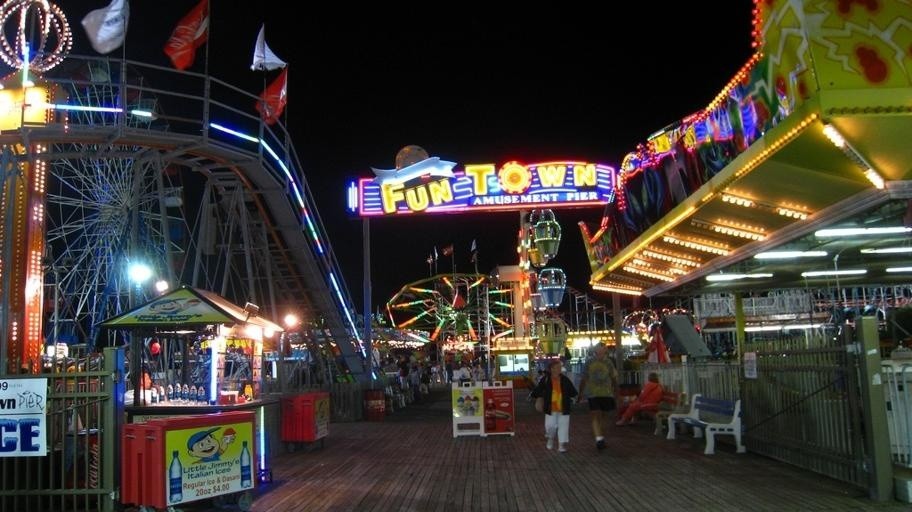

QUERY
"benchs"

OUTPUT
<box><xmin>631</xmin><ymin>391</ymin><xmax>689</xmax><ymax>436</ymax></box>
<box><xmin>666</xmin><ymin>394</ymin><xmax>747</xmax><ymax>456</ymax></box>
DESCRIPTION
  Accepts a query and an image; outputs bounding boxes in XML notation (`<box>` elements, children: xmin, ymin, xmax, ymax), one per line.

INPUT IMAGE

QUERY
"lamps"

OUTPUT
<box><xmin>244</xmin><ymin>302</ymin><xmax>259</xmax><ymax>323</ymax></box>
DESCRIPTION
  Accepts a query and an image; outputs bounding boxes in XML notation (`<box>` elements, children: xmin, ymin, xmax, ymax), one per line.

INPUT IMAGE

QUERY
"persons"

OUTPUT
<box><xmin>123</xmin><ymin>371</ymin><xmax>165</xmax><ymax>405</ymax></box>
<box><xmin>578</xmin><ymin>343</ymin><xmax>621</xmax><ymax>449</ymax></box>
<box><xmin>526</xmin><ymin>370</ymin><xmax>548</xmax><ymax>402</ymax></box>
<box><xmin>615</xmin><ymin>372</ymin><xmax>664</xmax><ymax>426</ymax></box>
<box><xmin>529</xmin><ymin>361</ymin><xmax>579</xmax><ymax>453</ymax></box>
<box><xmin>396</xmin><ymin>354</ymin><xmax>479</xmax><ymax>400</ymax></box>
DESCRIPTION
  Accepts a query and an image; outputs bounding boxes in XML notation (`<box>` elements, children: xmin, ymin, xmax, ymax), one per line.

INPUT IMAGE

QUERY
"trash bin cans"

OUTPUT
<box><xmin>616</xmin><ymin>385</ymin><xmax>640</xmax><ymax>422</ymax></box>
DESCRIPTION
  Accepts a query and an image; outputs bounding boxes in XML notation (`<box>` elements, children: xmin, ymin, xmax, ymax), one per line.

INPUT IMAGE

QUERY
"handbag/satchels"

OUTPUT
<box><xmin>535</xmin><ymin>397</ymin><xmax>545</xmax><ymax>413</ymax></box>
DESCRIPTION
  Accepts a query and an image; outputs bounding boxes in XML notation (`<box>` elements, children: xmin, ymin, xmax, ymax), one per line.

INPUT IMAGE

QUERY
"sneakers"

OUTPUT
<box><xmin>596</xmin><ymin>438</ymin><xmax>605</xmax><ymax>449</ymax></box>
<box><xmin>546</xmin><ymin>438</ymin><xmax>553</xmax><ymax>450</ymax></box>
<box><xmin>559</xmin><ymin>442</ymin><xmax>568</xmax><ymax>452</ymax></box>
<box><xmin>615</xmin><ymin>417</ymin><xmax>639</xmax><ymax>426</ymax></box>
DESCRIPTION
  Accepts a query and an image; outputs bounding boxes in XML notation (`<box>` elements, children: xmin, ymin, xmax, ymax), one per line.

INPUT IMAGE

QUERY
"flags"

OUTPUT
<box><xmin>255</xmin><ymin>67</ymin><xmax>288</xmax><ymax>126</ymax></box>
<box><xmin>251</xmin><ymin>23</ymin><xmax>287</xmax><ymax>71</ymax></box>
<box><xmin>427</xmin><ymin>240</ymin><xmax>478</xmax><ymax>262</ymax></box>
<box><xmin>164</xmin><ymin>0</ymin><xmax>210</xmax><ymax>70</ymax></box>
<box><xmin>82</xmin><ymin>0</ymin><xmax>130</xmax><ymax>55</ymax></box>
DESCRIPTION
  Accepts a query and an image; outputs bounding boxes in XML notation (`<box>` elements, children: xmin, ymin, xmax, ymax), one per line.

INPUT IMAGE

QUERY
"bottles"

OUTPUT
<box><xmin>151</xmin><ymin>383</ymin><xmax>206</xmax><ymax>405</ymax></box>
<box><xmin>169</xmin><ymin>450</ymin><xmax>182</xmax><ymax>503</ymax></box>
<box><xmin>240</xmin><ymin>440</ymin><xmax>252</xmax><ymax>488</ymax></box>
<box><xmin>485</xmin><ymin>398</ymin><xmax>496</xmax><ymax>433</ymax></box>
<box><xmin>245</xmin><ymin>384</ymin><xmax>253</xmax><ymax>402</ymax></box>
<box><xmin>457</xmin><ymin>395</ymin><xmax>480</xmax><ymax>417</ymax></box>
